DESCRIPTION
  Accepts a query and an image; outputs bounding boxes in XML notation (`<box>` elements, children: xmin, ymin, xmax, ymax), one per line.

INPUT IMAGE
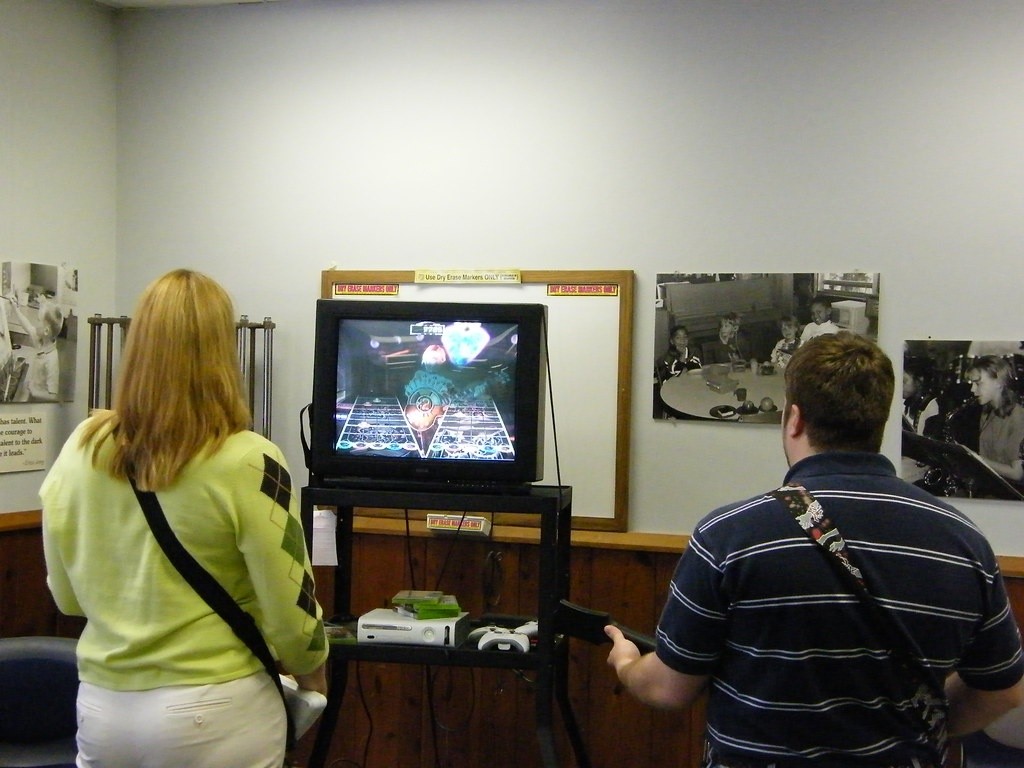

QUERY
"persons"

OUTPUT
<box><xmin>38</xmin><ymin>268</ymin><xmax>329</xmax><ymax>768</ymax></box>
<box><xmin>799</xmin><ymin>295</ymin><xmax>839</xmax><ymax>342</ymax></box>
<box><xmin>964</xmin><ymin>355</ymin><xmax>1024</xmax><ymax>481</ymax></box>
<box><xmin>902</xmin><ymin>358</ymin><xmax>944</xmax><ymax>441</ymax></box>
<box><xmin>9</xmin><ymin>294</ymin><xmax>64</xmax><ymax>402</ymax></box>
<box><xmin>770</xmin><ymin>315</ymin><xmax>804</xmax><ymax>368</ymax></box>
<box><xmin>662</xmin><ymin>324</ymin><xmax>703</xmax><ymax>381</ymax></box>
<box><xmin>713</xmin><ymin>311</ymin><xmax>751</xmax><ymax>363</ymax></box>
<box><xmin>604</xmin><ymin>329</ymin><xmax>1024</xmax><ymax>768</ymax></box>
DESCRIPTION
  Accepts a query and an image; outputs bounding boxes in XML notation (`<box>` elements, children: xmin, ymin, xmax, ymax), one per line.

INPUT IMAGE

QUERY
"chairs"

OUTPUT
<box><xmin>1</xmin><ymin>636</ymin><xmax>83</xmax><ymax>768</ymax></box>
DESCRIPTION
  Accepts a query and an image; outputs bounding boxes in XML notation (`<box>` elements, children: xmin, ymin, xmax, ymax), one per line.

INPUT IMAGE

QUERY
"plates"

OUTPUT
<box><xmin>736</xmin><ymin>406</ymin><xmax>759</xmax><ymax>416</ymax></box>
<box><xmin>759</xmin><ymin>405</ymin><xmax>778</xmax><ymax>413</ymax></box>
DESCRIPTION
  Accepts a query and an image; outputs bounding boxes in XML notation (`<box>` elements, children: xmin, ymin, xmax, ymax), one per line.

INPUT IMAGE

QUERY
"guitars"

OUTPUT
<box><xmin>542</xmin><ymin>597</ymin><xmax>659</xmax><ymax>659</ymax></box>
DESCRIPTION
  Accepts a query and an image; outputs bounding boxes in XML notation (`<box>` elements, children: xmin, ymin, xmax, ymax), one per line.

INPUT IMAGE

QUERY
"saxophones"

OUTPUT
<box><xmin>923</xmin><ymin>395</ymin><xmax>979</xmax><ymax>498</ymax></box>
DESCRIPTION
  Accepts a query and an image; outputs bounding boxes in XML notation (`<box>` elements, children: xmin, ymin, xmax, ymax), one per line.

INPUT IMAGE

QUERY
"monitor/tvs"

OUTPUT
<box><xmin>309</xmin><ymin>299</ymin><xmax>548</xmax><ymax>495</ymax></box>
